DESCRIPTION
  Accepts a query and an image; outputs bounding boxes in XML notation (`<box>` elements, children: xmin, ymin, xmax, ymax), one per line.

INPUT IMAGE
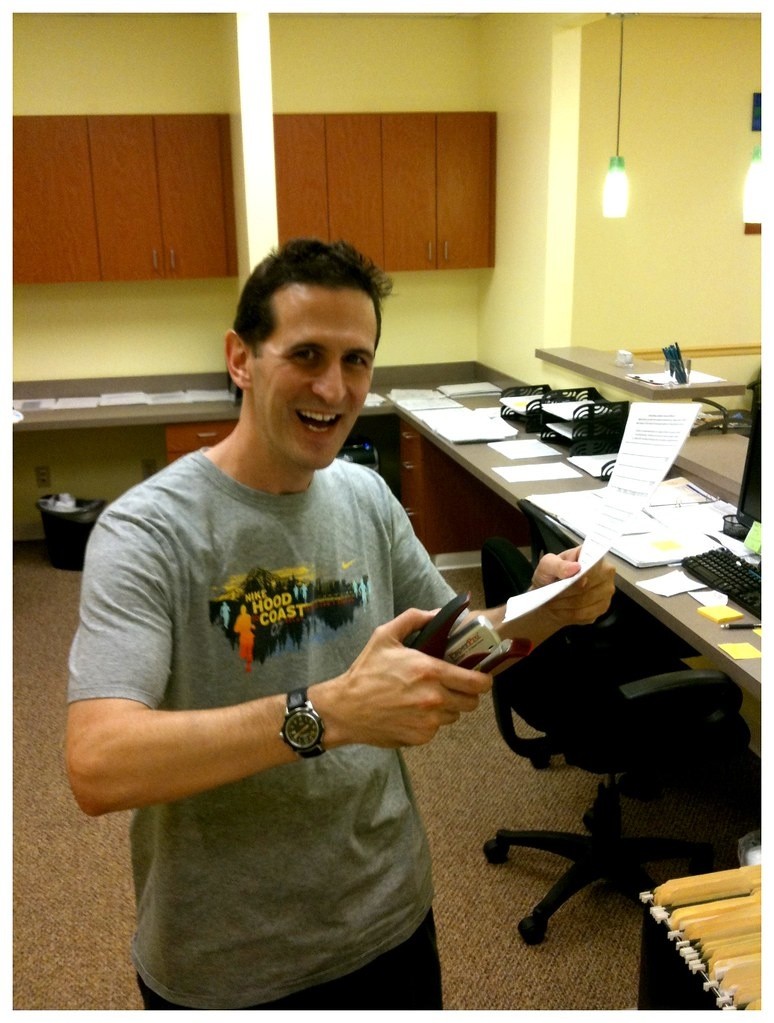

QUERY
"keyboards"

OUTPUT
<box><xmin>682</xmin><ymin>547</ymin><xmax>761</xmax><ymax>621</ymax></box>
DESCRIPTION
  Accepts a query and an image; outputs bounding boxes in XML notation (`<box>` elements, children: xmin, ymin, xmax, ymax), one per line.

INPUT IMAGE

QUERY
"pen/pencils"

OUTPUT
<box><xmin>663</xmin><ymin>343</ymin><xmax>690</xmax><ymax>386</ymax></box>
<box><xmin>725</xmin><ymin>624</ymin><xmax>761</xmax><ymax>632</ymax></box>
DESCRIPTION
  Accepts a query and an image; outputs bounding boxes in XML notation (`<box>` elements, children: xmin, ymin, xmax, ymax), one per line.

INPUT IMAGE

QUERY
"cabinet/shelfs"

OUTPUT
<box><xmin>275</xmin><ymin>110</ymin><xmax>498</xmax><ymax>270</ymax></box>
<box><xmin>13</xmin><ymin>114</ymin><xmax>239</xmax><ymax>284</ymax></box>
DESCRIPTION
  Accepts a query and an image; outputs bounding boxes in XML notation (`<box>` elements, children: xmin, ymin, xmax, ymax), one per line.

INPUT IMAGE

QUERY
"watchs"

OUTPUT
<box><xmin>277</xmin><ymin>687</ymin><xmax>326</xmax><ymax>760</ymax></box>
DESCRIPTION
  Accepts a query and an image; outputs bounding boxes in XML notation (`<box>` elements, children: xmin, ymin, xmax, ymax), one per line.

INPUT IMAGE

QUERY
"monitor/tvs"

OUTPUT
<box><xmin>736</xmin><ymin>383</ymin><xmax>761</xmax><ymax>529</ymax></box>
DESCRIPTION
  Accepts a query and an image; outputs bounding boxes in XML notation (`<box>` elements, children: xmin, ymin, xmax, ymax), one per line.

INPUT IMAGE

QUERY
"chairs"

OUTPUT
<box><xmin>480</xmin><ymin>500</ymin><xmax>751</xmax><ymax>945</ymax></box>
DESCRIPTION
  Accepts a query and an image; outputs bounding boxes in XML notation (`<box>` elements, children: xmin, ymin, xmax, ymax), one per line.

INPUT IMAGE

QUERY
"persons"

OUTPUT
<box><xmin>55</xmin><ymin>233</ymin><xmax>615</xmax><ymax>1010</ymax></box>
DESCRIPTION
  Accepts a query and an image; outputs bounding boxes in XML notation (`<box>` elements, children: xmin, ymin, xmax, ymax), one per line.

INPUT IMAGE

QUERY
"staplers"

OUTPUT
<box><xmin>404</xmin><ymin>588</ymin><xmax>529</xmax><ymax>672</ymax></box>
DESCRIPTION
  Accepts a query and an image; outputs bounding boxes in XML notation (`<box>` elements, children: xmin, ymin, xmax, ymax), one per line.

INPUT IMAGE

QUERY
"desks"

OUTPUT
<box><xmin>12</xmin><ymin>345</ymin><xmax>761</xmax><ymax>702</ymax></box>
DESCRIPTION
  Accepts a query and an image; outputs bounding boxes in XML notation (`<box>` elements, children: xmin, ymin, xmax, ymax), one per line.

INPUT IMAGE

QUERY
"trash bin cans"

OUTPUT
<box><xmin>35</xmin><ymin>492</ymin><xmax>106</xmax><ymax>569</ymax></box>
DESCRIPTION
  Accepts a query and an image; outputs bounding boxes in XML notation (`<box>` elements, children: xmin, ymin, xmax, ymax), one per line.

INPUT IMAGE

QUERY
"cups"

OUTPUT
<box><xmin>665</xmin><ymin>359</ymin><xmax>693</xmax><ymax>386</ymax></box>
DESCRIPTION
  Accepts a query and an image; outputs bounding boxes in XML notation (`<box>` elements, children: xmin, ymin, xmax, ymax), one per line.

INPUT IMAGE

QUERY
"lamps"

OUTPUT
<box><xmin>603</xmin><ymin>14</ymin><xmax>629</xmax><ymax>218</ymax></box>
<box><xmin>744</xmin><ymin>145</ymin><xmax>761</xmax><ymax>234</ymax></box>
